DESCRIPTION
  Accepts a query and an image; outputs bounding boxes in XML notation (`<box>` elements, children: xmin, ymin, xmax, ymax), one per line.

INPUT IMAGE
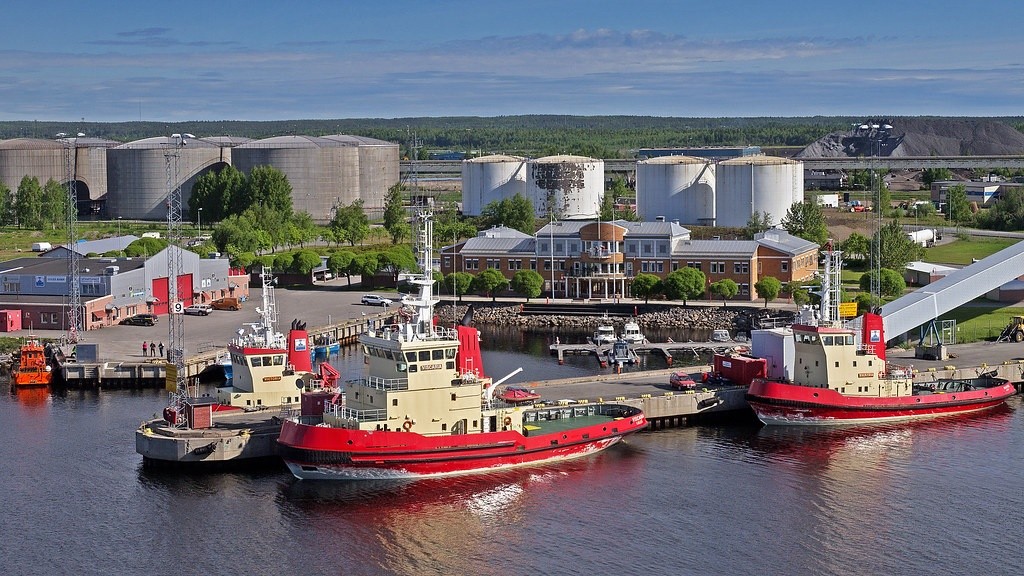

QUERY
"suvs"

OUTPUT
<box><xmin>361</xmin><ymin>295</ymin><xmax>394</xmax><ymax>307</ymax></box>
<box><xmin>184</xmin><ymin>304</ymin><xmax>213</xmax><ymax>316</ymax></box>
<box><xmin>124</xmin><ymin>313</ymin><xmax>159</xmax><ymax>326</ymax></box>
<box><xmin>670</xmin><ymin>372</ymin><xmax>696</xmax><ymax>390</ymax></box>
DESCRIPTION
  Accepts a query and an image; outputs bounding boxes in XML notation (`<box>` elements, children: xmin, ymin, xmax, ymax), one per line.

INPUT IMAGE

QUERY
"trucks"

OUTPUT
<box><xmin>210</xmin><ymin>298</ymin><xmax>241</xmax><ymax>311</ymax></box>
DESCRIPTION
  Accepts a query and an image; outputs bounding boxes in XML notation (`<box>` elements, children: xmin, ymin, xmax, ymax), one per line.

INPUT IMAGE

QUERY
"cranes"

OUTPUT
<box><xmin>992</xmin><ymin>316</ymin><xmax>1024</xmax><ymax>344</ymax></box>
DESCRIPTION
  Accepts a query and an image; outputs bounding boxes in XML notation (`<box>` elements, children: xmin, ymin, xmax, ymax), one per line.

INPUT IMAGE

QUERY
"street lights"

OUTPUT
<box><xmin>118</xmin><ymin>216</ymin><xmax>123</xmax><ymax>256</ymax></box>
<box><xmin>198</xmin><ymin>207</ymin><xmax>202</xmax><ymax>244</ymax></box>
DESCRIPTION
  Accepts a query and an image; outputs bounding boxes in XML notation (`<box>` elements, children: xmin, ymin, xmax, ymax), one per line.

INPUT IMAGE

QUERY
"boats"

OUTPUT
<box><xmin>274</xmin><ymin>122</ymin><xmax>649</xmax><ymax>480</ymax></box>
<box><xmin>608</xmin><ymin>335</ymin><xmax>635</xmax><ymax>365</ymax></box>
<box><xmin>620</xmin><ymin>315</ymin><xmax>644</xmax><ymax>344</ymax></box>
<box><xmin>313</xmin><ymin>327</ymin><xmax>340</xmax><ymax>352</ymax></box>
<box><xmin>747</xmin><ymin>231</ymin><xmax>1019</xmax><ymax>427</ymax></box>
<box><xmin>10</xmin><ymin>321</ymin><xmax>53</xmax><ymax>384</ymax></box>
<box><xmin>710</xmin><ymin>322</ymin><xmax>747</xmax><ymax>342</ymax></box>
<box><xmin>497</xmin><ymin>386</ymin><xmax>540</xmax><ymax>403</ymax></box>
<box><xmin>593</xmin><ymin>309</ymin><xmax>618</xmax><ymax>346</ymax></box>
<box><xmin>204</xmin><ymin>262</ymin><xmax>339</xmax><ymax>417</ymax></box>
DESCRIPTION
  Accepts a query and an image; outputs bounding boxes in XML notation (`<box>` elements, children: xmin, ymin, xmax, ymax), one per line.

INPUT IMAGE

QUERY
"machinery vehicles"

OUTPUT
<box><xmin>850</xmin><ymin>203</ymin><xmax>866</xmax><ymax>213</ymax></box>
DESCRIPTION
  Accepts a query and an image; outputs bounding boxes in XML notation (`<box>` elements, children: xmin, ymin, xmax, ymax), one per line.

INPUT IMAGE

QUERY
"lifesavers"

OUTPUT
<box><xmin>35</xmin><ymin>358</ymin><xmax>42</xmax><ymax>365</ymax></box>
<box><xmin>504</xmin><ymin>417</ymin><xmax>511</xmax><ymax>425</ymax></box>
<box><xmin>403</xmin><ymin>421</ymin><xmax>411</xmax><ymax>429</ymax></box>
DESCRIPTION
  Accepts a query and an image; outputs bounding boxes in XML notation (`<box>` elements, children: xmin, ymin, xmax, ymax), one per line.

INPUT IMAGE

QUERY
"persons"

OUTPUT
<box><xmin>150</xmin><ymin>343</ymin><xmax>155</xmax><ymax>357</ymax></box>
<box><xmin>158</xmin><ymin>341</ymin><xmax>164</xmax><ymax>357</ymax></box>
<box><xmin>71</xmin><ymin>346</ymin><xmax>76</xmax><ymax>357</ymax></box>
<box><xmin>143</xmin><ymin>342</ymin><xmax>148</xmax><ymax>356</ymax></box>
